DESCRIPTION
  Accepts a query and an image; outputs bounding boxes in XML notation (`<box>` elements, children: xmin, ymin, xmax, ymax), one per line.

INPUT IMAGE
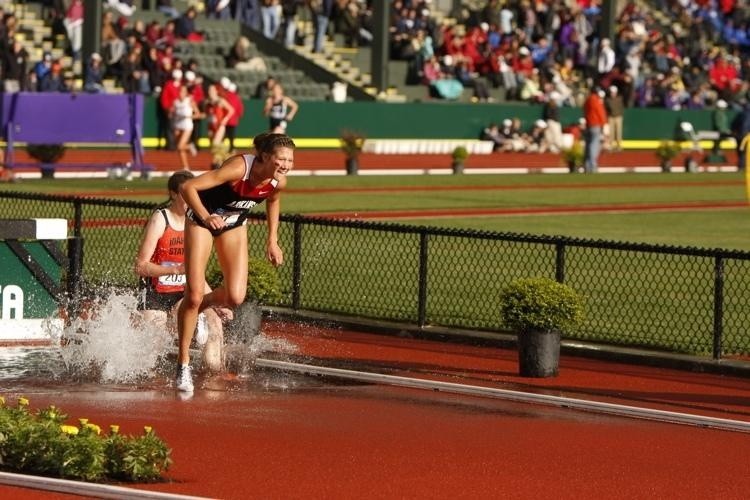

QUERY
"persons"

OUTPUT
<box><xmin>255</xmin><ymin>76</ymin><xmax>278</xmax><ymax>98</ymax></box>
<box><xmin>61</xmin><ymin>2</ymin><xmax>246</xmax><ymax>170</ymax></box>
<box><xmin>225</xmin><ymin>36</ymin><xmax>267</xmax><ymax>72</ymax></box>
<box><xmin>711</xmin><ymin>100</ymin><xmax>733</xmax><ymax>157</ymax></box>
<box><xmin>387</xmin><ymin>1</ymin><xmax>750</xmax><ymax>111</ymax></box>
<box><xmin>481</xmin><ymin>86</ymin><xmax>625</xmax><ymax>174</ymax></box>
<box><xmin>134</xmin><ymin>168</ymin><xmax>232</xmax><ymax>370</ymax></box>
<box><xmin>264</xmin><ymin>85</ymin><xmax>299</xmax><ymax>132</ymax></box>
<box><xmin>205</xmin><ymin>2</ymin><xmax>378</xmax><ymax>53</ymax></box>
<box><xmin>175</xmin><ymin>133</ymin><xmax>295</xmax><ymax>393</ymax></box>
<box><xmin>731</xmin><ymin>93</ymin><xmax>750</xmax><ymax>171</ymax></box>
<box><xmin>2</xmin><ymin>12</ymin><xmax>72</xmax><ymax>93</ymax></box>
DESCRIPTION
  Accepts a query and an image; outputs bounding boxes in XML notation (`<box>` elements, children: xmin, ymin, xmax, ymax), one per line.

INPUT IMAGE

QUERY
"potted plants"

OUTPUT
<box><xmin>502</xmin><ymin>277</ymin><xmax>583</xmax><ymax>377</ymax></box>
<box><xmin>204</xmin><ymin>255</ymin><xmax>280</xmax><ymax>346</ymax></box>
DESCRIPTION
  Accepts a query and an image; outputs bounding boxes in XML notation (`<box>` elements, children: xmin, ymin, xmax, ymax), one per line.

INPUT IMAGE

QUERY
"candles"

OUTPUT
<box><xmin>653</xmin><ymin>139</ymin><xmax>680</xmax><ymax>177</ymax></box>
<box><xmin>558</xmin><ymin>142</ymin><xmax>588</xmax><ymax>175</ymax></box>
<box><xmin>452</xmin><ymin>146</ymin><xmax>468</xmax><ymax>176</ymax></box>
<box><xmin>25</xmin><ymin>142</ymin><xmax>67</xmax><ymax>176</ymax></box>
<box><xmin>340</xmin><ymin>134</ymin><xmax>366</xmax><ymax>176</ymax></box>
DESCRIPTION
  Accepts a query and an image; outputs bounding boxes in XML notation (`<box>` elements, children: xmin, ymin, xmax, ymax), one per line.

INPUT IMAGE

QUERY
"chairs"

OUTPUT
<box><xmin>1</xmin><ymin>1</ymin><xmax>585</xmax><ymax>108</ymax></box>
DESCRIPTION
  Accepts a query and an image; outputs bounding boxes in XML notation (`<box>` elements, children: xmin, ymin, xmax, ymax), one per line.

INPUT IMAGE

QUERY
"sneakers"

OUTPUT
<box><xmin>175</xmin><ymin>364</ymin><xmax>193</xmax><ymax>392</ymax></box>
<box><xmin>194</xmin><ymin>312</ymin><xmax>208</xmax><ymax>345</ymax></box>
